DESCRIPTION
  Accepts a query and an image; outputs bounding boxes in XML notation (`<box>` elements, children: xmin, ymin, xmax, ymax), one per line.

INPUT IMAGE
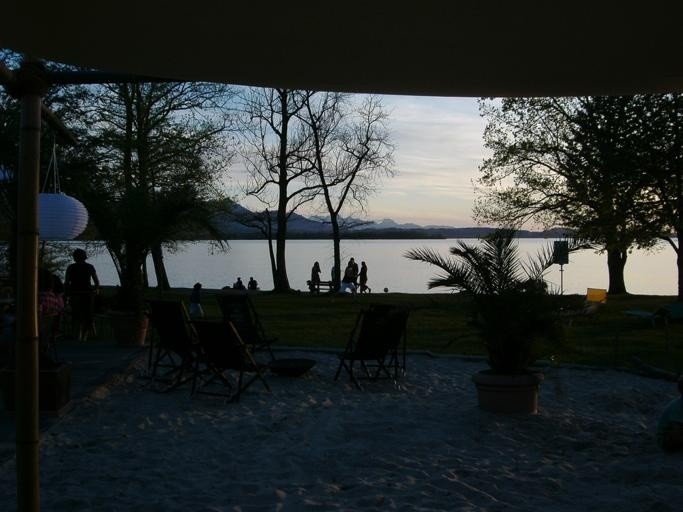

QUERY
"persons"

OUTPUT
<box><xmin>328</xmin><ymin>260</ymin><xmax>360</xmax><ymax>303</ymax></box>
<box><xmin>310</xmin><ymin>261</ymin><xmax>321</xmax><ymax>294</ymax></box>
<box><xmin>188</xmin><ymin>283</ymin><xmax>204</xmax><ymax>316</ymax></box>
<box><xmin>235</xmin><ymin>277</ymin><xmax>242</xmax><ymax>289</ymax></box>
<box><xmin>356</xmin><ymin>261</ymin><xmax>371</xmax><ymax>294</ymax></box>
<box><xmin>348</xmin><ymin>257</ymin><xmax>358</xmax><ymax>294</ymax></box>
<box><xmin>247</xmin><ymin>277</ymin><xmax>258</xmax><ymax>290</ymax></box>
<box><xmin>653</xmin><ymin>379</ymin><xmax>683</xmax><ymax>453</ymax></box>
<box><xmin>64</xmin><ymin>247</ymin><xmax>100</xmax><ymax>321</ymax></box>
<box><xmin>37</xmin><ymin>274</ymin><xmax>65</xmax><ymax>318</ymax></box>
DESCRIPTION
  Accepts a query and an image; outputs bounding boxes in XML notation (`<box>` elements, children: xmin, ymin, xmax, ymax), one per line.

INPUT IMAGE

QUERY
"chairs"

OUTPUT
<box><xmin>134</xmin><ymin>289</ymin><xmax>318</xmax><ymax>401</ymax></box>
<box><xmin>331</xmin><ymin>302</ymin><xmax>411</xmax><ymax>391</ymax></box>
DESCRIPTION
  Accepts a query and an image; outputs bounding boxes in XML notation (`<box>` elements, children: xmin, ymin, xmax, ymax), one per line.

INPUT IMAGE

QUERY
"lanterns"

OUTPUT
<box><xmin>37</xmin><ymin>191</ymin><xmax>88</xmax><ymax>240</ymax></box>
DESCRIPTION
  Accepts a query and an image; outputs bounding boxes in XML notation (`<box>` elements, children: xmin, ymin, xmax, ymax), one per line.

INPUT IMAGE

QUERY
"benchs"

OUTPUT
<box><xmin>307</xmin><ymin>280</ymin><xmax>336</xmax><ymax>292</ymax></box>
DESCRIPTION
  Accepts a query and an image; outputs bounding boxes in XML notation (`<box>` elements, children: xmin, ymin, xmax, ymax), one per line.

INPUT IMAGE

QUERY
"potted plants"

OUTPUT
<box><xmin>411</xmin><ymin>228</ymin><xmax>585</xmax><ymax>414</ymax></box>
<box><xmin>106</xmin><ymin>286</ymin><xmax>148</xmax><ymax>350</ymax></box>
<box><xmin>0</xmin><ymin>333</ymin><xmax>75</xmax><ymax>419</ymax></box>
